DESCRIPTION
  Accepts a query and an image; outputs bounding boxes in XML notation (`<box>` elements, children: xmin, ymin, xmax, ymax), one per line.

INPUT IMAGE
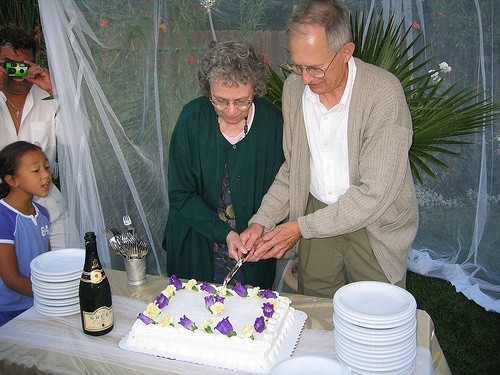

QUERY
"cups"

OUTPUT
<box><xmin>123</xmin><ymin>258</ymin><xmax>146</xmax><ymax>287</ymax></box>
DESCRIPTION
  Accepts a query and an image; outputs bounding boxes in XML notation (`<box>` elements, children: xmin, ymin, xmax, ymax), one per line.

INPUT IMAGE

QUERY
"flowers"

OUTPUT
<box><xmin>136</xmin><ymin>274</ymin><xmax>279</xmax><ymax>338</ymax></box>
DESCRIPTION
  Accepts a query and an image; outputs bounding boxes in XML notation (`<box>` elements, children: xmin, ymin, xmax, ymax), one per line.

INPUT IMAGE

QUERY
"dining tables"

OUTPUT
<box><xmin>0</xmin><ymin>268</ymin><xmax>451</xmax><ymax>375</ymax></box>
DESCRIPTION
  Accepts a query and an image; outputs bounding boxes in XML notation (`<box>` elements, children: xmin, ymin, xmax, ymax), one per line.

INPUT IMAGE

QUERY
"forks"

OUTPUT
<box><xmin>99</xmin><ymin>214</ymin><xmax>150</xmax><ymax>259</ymax></box>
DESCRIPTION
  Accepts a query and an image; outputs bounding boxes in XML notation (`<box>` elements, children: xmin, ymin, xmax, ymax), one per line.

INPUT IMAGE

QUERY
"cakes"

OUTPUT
<box><xmin>126</xmin><ymin>274</ymin><xmax>296</xmax><ymax>371</ymax></box>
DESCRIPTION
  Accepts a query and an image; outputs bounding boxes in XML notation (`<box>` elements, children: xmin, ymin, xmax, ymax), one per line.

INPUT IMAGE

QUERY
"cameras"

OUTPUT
<box><xmin>4</xmin><ymin>60</ymin><xmax>28</xmax><ymax>77</ymax></box>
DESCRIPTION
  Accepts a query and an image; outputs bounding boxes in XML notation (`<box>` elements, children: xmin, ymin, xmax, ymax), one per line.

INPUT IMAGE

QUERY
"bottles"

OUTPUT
<box><xmin>79</xmin><ymin>232</ymin><xmax>114</xmax><ymax>337</ymax></box>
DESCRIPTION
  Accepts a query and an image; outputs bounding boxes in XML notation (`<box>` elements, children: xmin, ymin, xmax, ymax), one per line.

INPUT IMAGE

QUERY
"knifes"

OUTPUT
<box><xmin>223</xmin><ymin>250</ymin><xmax>251</xmax><ymax>286</ymax></box>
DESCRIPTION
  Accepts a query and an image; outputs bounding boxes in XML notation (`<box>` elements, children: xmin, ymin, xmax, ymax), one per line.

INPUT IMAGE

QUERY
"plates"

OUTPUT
<box><xmin>266</xmin><ymin>355</ymin><xmax>350</xmax><ymax>375</ymax></box>
<box><xmin>333</xmin><ymin>280</ymin><xmax>416</xmax><ymax>375</ymax></box>
<box><xmin>30</xmin><ymin>247</ymin><xmax>86</xmax><ymax>317</ymax></box>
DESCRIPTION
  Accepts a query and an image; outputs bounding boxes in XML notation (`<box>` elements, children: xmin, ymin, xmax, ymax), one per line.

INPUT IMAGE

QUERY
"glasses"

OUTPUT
<box><xmin>284</xmin><ymin>48</ymin><xmax>342</xmax><ymax>77</ymax></box>
<box><xmin>209</xmin><ymin>94</ymin><xmax>253</xmax><ymax>110</ymax></box>
